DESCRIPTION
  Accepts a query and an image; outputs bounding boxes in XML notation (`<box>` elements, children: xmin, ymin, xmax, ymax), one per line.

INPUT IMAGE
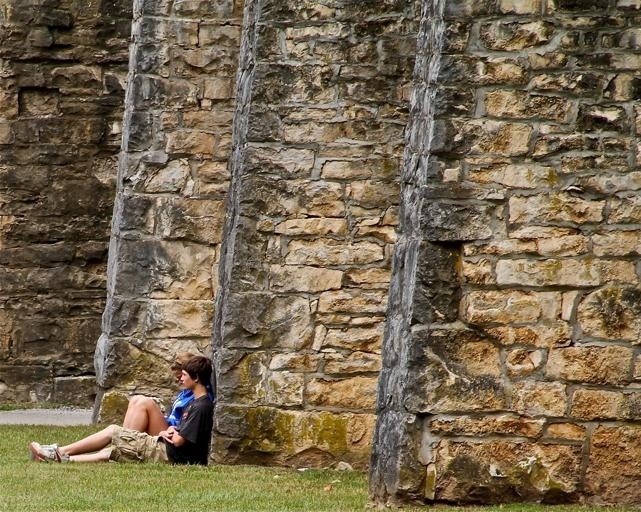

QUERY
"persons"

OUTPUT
<box><xmin>31</xmin><ymin>355</ymin><xmax>215</xmax><ymax>466</ymax></box>
<box><xmin>122</xmin><ymin>353</ymin><xmax>195</xmax><ymax>437</ymax></box>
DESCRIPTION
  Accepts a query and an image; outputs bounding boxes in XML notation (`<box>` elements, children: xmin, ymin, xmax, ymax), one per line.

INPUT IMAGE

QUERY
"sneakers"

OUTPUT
<box><xmin>31</xmin><ymin>441</ymin><xmax>69</xmax><ymax>463</ymax></box>
